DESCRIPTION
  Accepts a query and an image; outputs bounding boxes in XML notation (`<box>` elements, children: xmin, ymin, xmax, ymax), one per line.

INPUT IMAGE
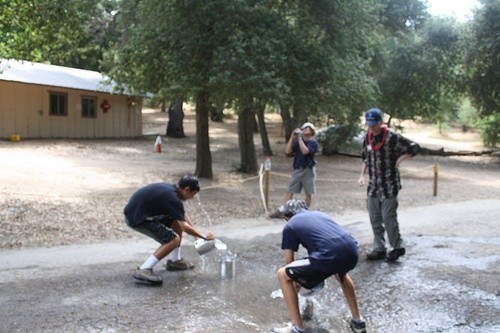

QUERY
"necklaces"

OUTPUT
<box><xmin>369</xmin><ymin>124</ymin><xmax>387</xmax><ymax>151</ymax></box>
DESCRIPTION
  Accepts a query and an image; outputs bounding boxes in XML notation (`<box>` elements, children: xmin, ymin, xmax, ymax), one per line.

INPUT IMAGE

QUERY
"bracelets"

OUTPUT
<box><xmin>202</xmin><ymin>233</ymin><xmax>206</xmax><ymax>240</ymax></box>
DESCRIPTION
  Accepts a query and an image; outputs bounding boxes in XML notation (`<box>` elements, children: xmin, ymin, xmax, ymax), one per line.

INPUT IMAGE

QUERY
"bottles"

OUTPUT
<box><xmin>264</xmin><ymin>157</ymin><xmax>271</xmax><ymax>170</ymax></box>
<box><xmin>155</xmin><ymin>135</ymin><xmax>161</xmax><ymax>152</ymax></box>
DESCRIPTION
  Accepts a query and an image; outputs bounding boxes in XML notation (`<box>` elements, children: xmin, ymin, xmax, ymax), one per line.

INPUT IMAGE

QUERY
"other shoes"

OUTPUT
<box><xmin>367</xmin><ymin>247</ymin><xmax>387</xmax><ymax>260</ymax></box>
<box><xmin>270</xmin><ymin>324</ymin><xmax>305</xmax><ymax>333</ymax></box>
<box><xmin>132</xmin><ymin>266</ymin><xmax>163</xmax><ymax>283</ymax></box>
<box><xmin>269</xmin><ymin>209</ymin><xmax>284</xmax><ymax>218</ymax></box>
<box><xmin>165</xmin><ymin>257</ymin><xmax>194</xmax><ymax>271</ymax></box>
<box><xmin>349</xmin><ymin>316</ymin><xmax>367</xmax><ymax>333</ymax></box>
<box><xmin>384</xmin><ymin>247</ymin><xmax>406</xmax><ymax>263</ymax></box>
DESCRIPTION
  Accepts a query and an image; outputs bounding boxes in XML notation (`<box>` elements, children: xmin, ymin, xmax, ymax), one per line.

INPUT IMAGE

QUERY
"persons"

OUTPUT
<box><xmin>270</xmin><ymin>199</ymin><xmax>366</xmax><ymax>333</ymax></box>
<box><xmin>269</xmin><ymin>122</ymin><xmax>318</xmax><ymax>218</ymax></box>
<box><xmin>123</xmin><ymin>174</ymin><xmax>214</xmax><ymax>284</ymax></box>
<box><xmin>358</xmin><ymin>109</ymin><xmax>421</xmax><ymax>261</ymax></box>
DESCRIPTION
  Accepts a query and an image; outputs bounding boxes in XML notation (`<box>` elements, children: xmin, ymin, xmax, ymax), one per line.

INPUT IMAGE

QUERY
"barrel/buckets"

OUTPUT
<box><xmin>221</xmin><ymin>260</ymin><xmax>235</xmax><ymax>279</ymax></box>
<box><xmin>194</xmin><ymin>237</ymin><xmax>216</xmax><ymax>255</ymax></box>
<box><xmin>299</xmin><ymin>279</ymin><xmax>324</xmax><ymax>296</ymax></box>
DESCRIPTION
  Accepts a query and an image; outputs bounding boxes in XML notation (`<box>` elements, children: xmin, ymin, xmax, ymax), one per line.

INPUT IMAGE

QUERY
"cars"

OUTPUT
<box><xmin>312</xmin><ymin>124</ymin><xmax>368</xmax><ymax>156</ymax></box>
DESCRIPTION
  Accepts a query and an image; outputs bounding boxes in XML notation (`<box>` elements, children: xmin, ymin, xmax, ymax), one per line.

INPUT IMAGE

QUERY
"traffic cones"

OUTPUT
<box><xmin>153</xmin><ymin>136</ymin><xmax>161</xmax><ymax>153</ymax></box>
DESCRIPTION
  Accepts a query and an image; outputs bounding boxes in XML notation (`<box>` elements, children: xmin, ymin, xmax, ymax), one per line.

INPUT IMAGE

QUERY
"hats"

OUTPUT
<box><xmin>301</xmin><ymin>122</ymin><xmax>315</xmax><ymax>131</ymax></box>
<box><xmin>285</xmin><ymin>198</ymin><xmax>309</xmax><ymax>214</ymax></box>
<box><xmin>365</xmin><ymin>109</ymin><xmax>384</xmax><ymax>126</ymax></box>
